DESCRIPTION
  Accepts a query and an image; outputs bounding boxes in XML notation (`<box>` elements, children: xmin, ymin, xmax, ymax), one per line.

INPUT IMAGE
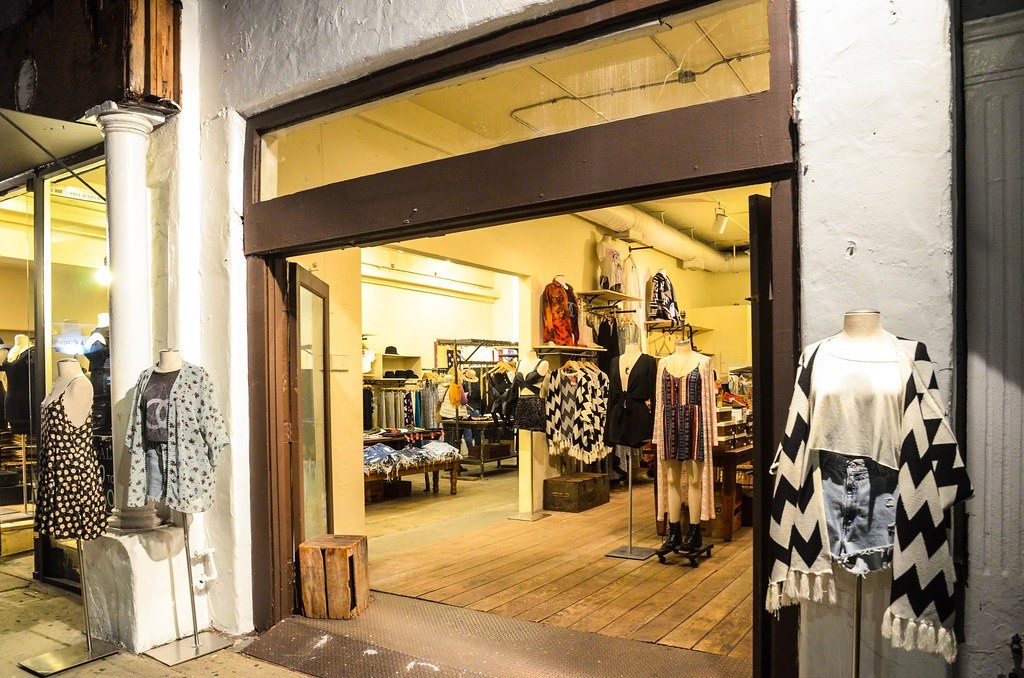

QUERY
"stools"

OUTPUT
<box><xmin>298</xmin><ymin>533</ymin><xmax>370</xmax><ymax>621</ymax></box>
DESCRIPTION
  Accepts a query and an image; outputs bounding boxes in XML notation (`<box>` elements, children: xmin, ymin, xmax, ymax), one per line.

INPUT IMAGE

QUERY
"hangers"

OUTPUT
<box><xmin>587</xmin><ymin>303</ymin><xmax>640</xmax><ymax>331</ymax></box>
<box><xmin>480</xmin><ymin>354</ymin><xmax>518</xmax><ymax>379</ymax></box>
<box><xmin>649</xmin><ymin>326</ymin><xmax>680</xmax><ymax>343</ymax></box>
<box><xmin>551</xmin><ymin>353</ymin><xmax>608</xmax><ymax>380</ymax></box>
<box><xmin>378</xmin><ymin>370</ymin><xmax>446</xmax><ymax>392</ymax></box>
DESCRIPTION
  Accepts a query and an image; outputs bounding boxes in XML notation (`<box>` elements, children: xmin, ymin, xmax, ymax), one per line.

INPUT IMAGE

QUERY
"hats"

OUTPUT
<box><xmin>406</xmin><ymin>370</ymin><xmax>418</xmax><ymax>378</ymax></box>
<box><xmin>385</xmin><ymin>346</ymin><xmax>397</xmax><ymax>354</ymax></box>
<box><xmin>448</xmin><ymin>367</ymin><xmax>460</xmax><ymax>376</ymax></box>
<box><xmin>463</xmin><ymin>369</ymin><xmax>479</xmax><ymax>383</ymax></box>
<box><xmin>395</xmin><ymin>371</ymin><xmax>406</xmax><ymax>377</ymax></box>
<box><xmin>383</xmin><ymin>371</ymin><xmax>395</xmax><ymax>377</ymax></box>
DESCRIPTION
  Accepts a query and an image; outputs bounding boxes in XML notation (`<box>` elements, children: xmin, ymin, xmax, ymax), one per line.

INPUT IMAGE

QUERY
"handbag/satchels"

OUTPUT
<box><xmin>435</xmin><ymin>403</ymin><xmax>442</xmax><ymax>423</ymax></box>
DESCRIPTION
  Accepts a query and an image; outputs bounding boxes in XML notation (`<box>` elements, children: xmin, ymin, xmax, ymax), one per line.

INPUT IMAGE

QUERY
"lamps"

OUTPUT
<box><xmin>711</xmin><ymin>201</ymin><xmax>729</xmax><ymax>235</ymax></box>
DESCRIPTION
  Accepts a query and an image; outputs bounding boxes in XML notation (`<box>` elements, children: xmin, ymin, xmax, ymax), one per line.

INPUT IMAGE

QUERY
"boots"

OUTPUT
<box><xmin>680</xmin><ymin>524</ymin><xmax>702</xmax><ymax>551</ymax></box>
<box><xmin>660</xmin><ymin>521</ymin><xmax>682</xmax><ymax>551</ymax></box>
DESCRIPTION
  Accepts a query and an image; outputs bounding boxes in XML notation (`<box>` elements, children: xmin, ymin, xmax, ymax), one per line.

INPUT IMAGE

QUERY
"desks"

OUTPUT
<box><xmin>439</xmin><ymin>416</ymin><xmax>519</xmax><ymax>482</ymax></box>
<box><xmin>363</xmin><ymin>457</ymin><xmax>460</xmax><ymax>496</ymax></box>
<box><xmin>713</xmin><ymin>444</ymin><xmax>753</xmax><ymax>542</ymax></box>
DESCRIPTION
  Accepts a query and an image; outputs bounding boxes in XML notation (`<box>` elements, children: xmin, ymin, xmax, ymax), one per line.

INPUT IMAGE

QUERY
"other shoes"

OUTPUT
<box><xmin>723</xmin><ymin>392</ymin><xmax>748</xmax><ymax>407</ymax></box>
<box><xmin>459</xmin><ymin>465</ymin><xmax>468</xmax><ymax>472</ymax></box>
<box><xmin>722</xmin><ymin>399</ymin><xmax>745</xmax><ymax>408</ymax></box>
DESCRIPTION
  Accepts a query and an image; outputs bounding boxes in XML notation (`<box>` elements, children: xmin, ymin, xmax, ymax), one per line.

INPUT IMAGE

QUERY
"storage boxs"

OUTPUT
<box><xmin>469</xmin><ymin>442</ymin><xmax>511</xmax><ymax>460</ymax></box>
<box><xmin>665</xmin><ymin>479</ymin><xmax>742</xmax><ymax>539</ymax></box>
<box><xmin>542</xmin><ymin>472</ymin><xmax>612</xmax><ymax>513</ymax></box>
<box><xmin>715</xmin><ymin>406</ymin><xmax>753</xmax><ymax>451</ymax></box>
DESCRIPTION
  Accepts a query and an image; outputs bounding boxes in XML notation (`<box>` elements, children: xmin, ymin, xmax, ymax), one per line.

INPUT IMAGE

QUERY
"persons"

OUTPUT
<box><xmin>597</xmin><ymin>234</ymin><xmax>622</xmax><ymax>295</ymax></box>
<box><xmin>603</xmin><ymin>339</ymin><xmax>657</xmax><ymax>447</ymax></box>
<box><xmin>4</xmin><ymin>334</ymin><xmax>35</xmax><ymax>434</ymax></box>
<box><xmin>649</xmin><ymin>339</ymin><xmax>716</xmax><ymax>555</ymax></box>
<box><xmin>516</xmin><ymin>349</ymin><xmax>551</xmax><ymax>431</ymax></box>
<box><xmin>80</xmin><ymin>313</ymin><xmax>113</xmax><ymax>516</ymax></box>
<box><xmin>460</xmin><ymin>369</ymin><xmax>481</xmax><ymax>451</ymax></box>
<box><xmin>760</xmin><ymin>309</ymin><xmax>972</xmax><ymax>663</ymax></box>
<box><xmin>35</xmin><ymin>358</ymin><xmax>107</xmax><ymax>537</ymax></box>
<box><xmin>125</xmin><ymin>350</ymin><xmax>230</xmax><ymax>511</ymax></box>
<box><xmin>437</xmin><ymin>367</ymin><xmax>468</xmax><ymax>473</ymax></box>
